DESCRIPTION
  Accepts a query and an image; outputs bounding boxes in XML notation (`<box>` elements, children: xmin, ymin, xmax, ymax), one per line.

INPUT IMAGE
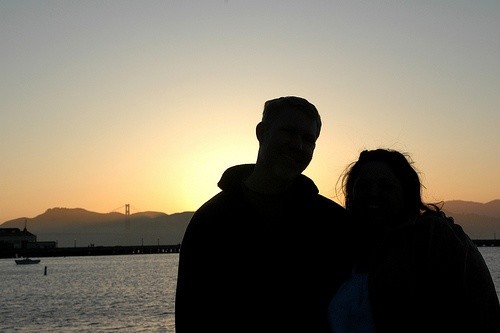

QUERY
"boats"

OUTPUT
<box><xmin>15</xmin><ymin>258</ymin><xmax>41</xmax><ymax>265</ymax></box>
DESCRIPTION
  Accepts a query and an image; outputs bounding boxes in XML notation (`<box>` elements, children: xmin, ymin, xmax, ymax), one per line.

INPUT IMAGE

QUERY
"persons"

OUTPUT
<box><xmin>177</xmin><ymin>95</ymin><xmax>456</xmax><ymax>333</ymax></box>
<box><xmin>322</xmin><ymin>149</ymin><xmax>500</xmax><ymax>333</ymax></box>
